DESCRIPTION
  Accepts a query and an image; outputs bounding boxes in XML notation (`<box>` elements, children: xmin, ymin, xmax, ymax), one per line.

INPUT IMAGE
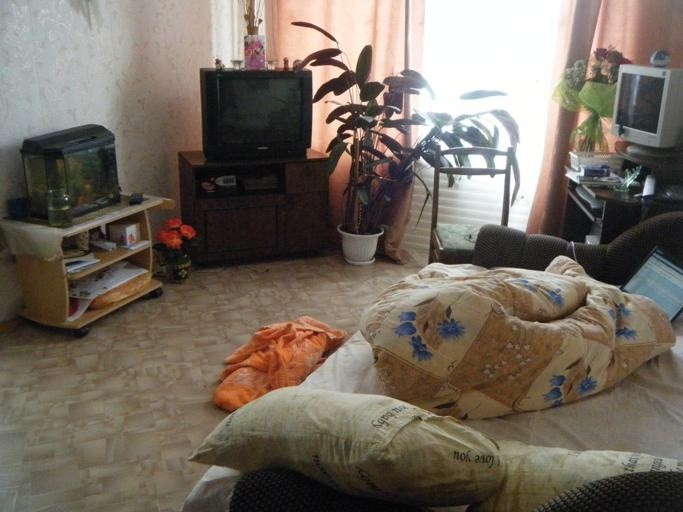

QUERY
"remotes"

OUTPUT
<box><xmin>129</xmin><ymin>193</ymin><xmax>142</xmax><ymax>204</ymax></box>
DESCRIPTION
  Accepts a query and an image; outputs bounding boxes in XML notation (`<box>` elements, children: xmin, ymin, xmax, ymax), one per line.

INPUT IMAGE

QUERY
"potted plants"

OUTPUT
<box><xmin>291</xmin><ymin>21</ymin><xmax>521</xmax><ymax>266</ymax></box>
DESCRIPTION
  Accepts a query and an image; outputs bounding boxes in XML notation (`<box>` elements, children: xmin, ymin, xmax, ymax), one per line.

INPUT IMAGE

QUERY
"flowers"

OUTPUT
<box><xmin>153</xmin><ymin>218</ymin><xmax>197</xmax><ymax>254</ymax></box>
<box><xmin>552</xmin><ymin>48</ymin><xmax>632</xmax><ymax>150</ymax></box>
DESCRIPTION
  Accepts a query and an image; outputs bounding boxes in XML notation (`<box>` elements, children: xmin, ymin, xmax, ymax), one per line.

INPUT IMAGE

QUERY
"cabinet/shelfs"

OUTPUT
<box><xmin>177</xmin><ymin>150</ymin><xmax>330</xmax><ymax>265</ymax></box>
<box><xmin>0</xmin><ymin>191</ymin><xmax>164</xmax><ymax>339</ymax></box>
<box><xmin>558</xmin><ymin>143</ymin><xmax>683</xmax><ymax>246</ymax></box>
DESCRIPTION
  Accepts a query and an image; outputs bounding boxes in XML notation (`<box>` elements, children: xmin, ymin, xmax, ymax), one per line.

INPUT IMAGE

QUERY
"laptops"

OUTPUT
<box><xmin>620</xmin><ymin>245</ymin><xmax>683</xmax><ymax>321</ymax></box>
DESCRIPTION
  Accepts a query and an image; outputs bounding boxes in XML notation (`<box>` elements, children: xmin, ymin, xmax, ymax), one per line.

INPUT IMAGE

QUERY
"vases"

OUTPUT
<box><xmin>165</xmin><ymin>254</ymin><xmax>191</xmax><ymax>284</ymax></box>
<box><xmin>244</xmin><ymin>34</ymin><xmax>266</xmax><ymax>70</ymax></box>
<box><xmin>569</xmin><ymin>114</ymin><xmax>609</xmax><ymax>153</ymax></box>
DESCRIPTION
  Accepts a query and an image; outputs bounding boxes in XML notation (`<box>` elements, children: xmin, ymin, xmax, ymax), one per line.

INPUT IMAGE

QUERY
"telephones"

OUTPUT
<box><xmin>642</xmin><ymin>174</ymin><xmax>656</xmax><ymax>200</ymax></box>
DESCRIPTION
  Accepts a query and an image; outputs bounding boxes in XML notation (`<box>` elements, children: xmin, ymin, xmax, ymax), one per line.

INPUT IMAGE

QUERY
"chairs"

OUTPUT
<box><xmin>428</xmin><ymin>147</ymin><xmax>513</xmax><ymax>265</ymax></box>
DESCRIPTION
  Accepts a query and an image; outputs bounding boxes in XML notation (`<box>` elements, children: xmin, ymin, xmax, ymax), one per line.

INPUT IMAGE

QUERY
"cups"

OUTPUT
<box><xmin>230</xmin><ymin>58</ymin><xmax>242</xmax><ymax>71</ymax></box>
<box><xmin>265</xmin><ymin>57</ymin><xmax>276</xmax><ymax>72</ymax></box>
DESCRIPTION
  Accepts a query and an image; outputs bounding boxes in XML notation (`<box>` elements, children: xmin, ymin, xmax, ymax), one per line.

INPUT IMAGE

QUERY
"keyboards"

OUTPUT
<box><xmin>576</xmin><ymin>184</ymin><xmax>603</xmax><ymax>209</ymax></box>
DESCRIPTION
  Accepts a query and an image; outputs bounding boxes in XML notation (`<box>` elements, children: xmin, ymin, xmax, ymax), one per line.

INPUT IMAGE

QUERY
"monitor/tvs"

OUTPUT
<box><xmin>612</xmin><ymin>65</ymin><xmax>682</xmax><ymax>158</ymax></box>
<box><xmin>200</xmin><ymin>68</ymin><xmax>312</xmax><ymax>159</ymax></box>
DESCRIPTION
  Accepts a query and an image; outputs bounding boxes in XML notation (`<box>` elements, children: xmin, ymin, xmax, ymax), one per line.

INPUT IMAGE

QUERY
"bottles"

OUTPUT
<box><xmin>214</xmin><ymin>57</ymin><xmax>222</xmax><ymax>69</ymax></box>
<box><xmin>46</xmin><ymin>186</ymin><xmax>71</xmax><ymax>228</ymax></box>
<box><xmin>282</xmin><ymin>57</ymin><xmax>288</xmax><ymax>70</ymax></box>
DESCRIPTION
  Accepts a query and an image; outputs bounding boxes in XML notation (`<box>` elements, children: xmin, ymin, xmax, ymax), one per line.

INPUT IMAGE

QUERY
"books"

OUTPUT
<box><xmin>563</xmin><ymin>164</ymin><xmax>624</xmax><ymax>186</ymax></box>
<box><xmin>576</xmin><ymin>163</ymin><xmax>611</xmax><ymax>177</ymax></box>
<box><xmin>63</xmin><ymin>255</ymin><xmax>100</xmax><ymax>275</ymax></box>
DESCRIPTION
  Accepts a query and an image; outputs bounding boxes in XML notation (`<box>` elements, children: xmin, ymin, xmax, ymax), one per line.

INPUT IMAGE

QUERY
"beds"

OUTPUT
<box><xmin>181</xmin><ymin>260</ymin><xmax>682</xmax><ymax>511</ymax></box>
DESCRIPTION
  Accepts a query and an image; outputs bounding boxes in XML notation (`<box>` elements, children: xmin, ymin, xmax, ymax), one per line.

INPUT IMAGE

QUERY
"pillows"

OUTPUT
<box><xmin>465</xmin><ymin>439</ymin><xmax>682</xmax><ymax>512</ymax></box>
<box><xmin>189</xmin><ymin>388</ymin><xmax>507</xmax><ymax>506</ymax></box>
